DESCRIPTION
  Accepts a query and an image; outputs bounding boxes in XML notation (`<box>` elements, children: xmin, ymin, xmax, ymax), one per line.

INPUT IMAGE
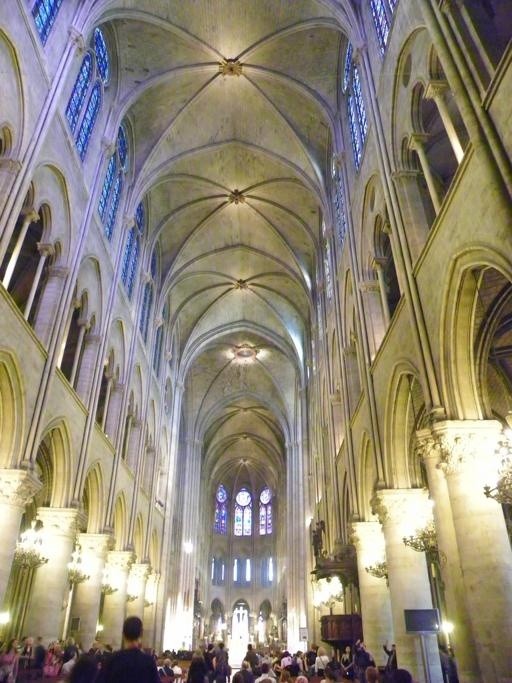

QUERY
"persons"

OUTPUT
<box><xmin>0</xmin><ymin>637</ymin><xmax>413</xmax><ymax>682</ymax></box>
<box><xmin>438</xmin><ymin>644</ymin><xmax>459</xmax><ymax>682</ymax></box>
<box><xmin>92</xmin><ymin>615</ymin><xmax>160</xmax><ymax>682</ymax></box>
